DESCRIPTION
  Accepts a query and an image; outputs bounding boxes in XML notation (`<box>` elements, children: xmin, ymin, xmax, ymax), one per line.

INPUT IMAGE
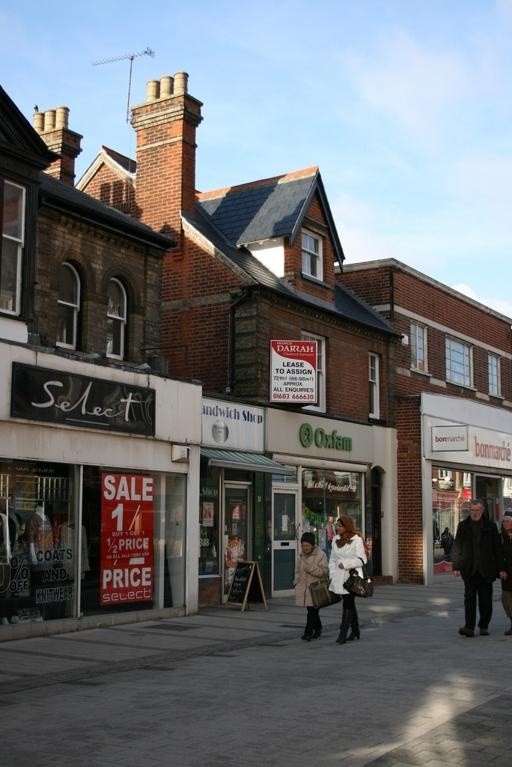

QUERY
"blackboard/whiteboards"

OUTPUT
<box><xmin>227</xmin><ymin>562</ymin><xmax>255</xmax><ymax>606</ymax></box>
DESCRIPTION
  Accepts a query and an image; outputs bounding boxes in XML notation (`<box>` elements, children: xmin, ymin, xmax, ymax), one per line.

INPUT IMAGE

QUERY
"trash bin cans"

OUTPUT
<box><xmin>37</xmin><ymin>580</ymin><xmax>73</xmax><ymax>620</ymax></box>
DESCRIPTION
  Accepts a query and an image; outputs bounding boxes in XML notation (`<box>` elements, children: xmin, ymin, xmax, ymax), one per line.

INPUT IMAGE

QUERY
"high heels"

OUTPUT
<box><xmin>301</xmin><ymin>630</ymin><xmax>322</xmax><ymax>641</ymax></box>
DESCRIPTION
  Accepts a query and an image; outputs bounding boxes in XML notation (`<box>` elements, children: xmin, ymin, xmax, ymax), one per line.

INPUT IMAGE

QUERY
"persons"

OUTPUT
<box><xmin>440</xmin><ymin>527</ymin><xmax>454</xmax><ymax>556</ymax></box>
<box><xmin>291</xmin><ymin>532</ymin><xmax>329</xmax><ymax>641</ymax></box>
<box><xmin>328</xmin><ymin>515</ymin><xmax>368</xmax><ymax>644</ymax></box>
<box><xmin>451</xmin><ymin>499</ymin><xmax>507</xmax><ymax>638</ymax></box>
<box><xmin>498</xmin><ymin>510</ymin><xmax>512</xmax><ymax>636</ymax></box>
<box><xmin>0</xmin><ymin>505</ymin><xmax>54</xmax><ymax>626</ymax></box>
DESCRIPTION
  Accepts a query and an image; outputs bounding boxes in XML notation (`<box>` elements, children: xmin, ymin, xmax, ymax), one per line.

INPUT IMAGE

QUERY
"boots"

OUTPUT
<box><xmin>335</xmin><ymin>609</ymin><xmax>360</xmax><ymax>644</ymax></box>
<box><xmin>504</xmin><ymin>616</ymin><xmax>512</xmax><ymax>635</ymax></box>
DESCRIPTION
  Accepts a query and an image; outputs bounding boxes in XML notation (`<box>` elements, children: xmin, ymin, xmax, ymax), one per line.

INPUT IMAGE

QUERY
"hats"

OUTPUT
<box><xmin>300</xmin><ymin>532</ymin><xmax>316</xmax><ymax>546</ymax></box>
<box><xmin>501</xmin><ymin>507</ymin><xmax>512</xmax><ymax>522</ymax></box>
<box><xmin>337</xmin><ymin>515</ymin><xmax>354</xmax><ymax>534</ymax></box>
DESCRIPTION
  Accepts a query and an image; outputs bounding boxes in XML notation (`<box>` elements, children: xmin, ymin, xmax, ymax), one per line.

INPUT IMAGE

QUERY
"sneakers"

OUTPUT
<box><xmin>480</xmin><ymin>627</ymin><xmax>489</xmax><ymax>636</ymax></box>
<box><xmin>459</xmin><ymin>624</ymin><xmax>474</xmax><ymax>637</ymax></box>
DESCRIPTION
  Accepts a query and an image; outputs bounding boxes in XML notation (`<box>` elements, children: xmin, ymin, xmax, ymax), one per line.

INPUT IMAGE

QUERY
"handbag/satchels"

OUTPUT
<box><xmin>308</xmin><ymin>567</ymin><xmax>342</xmax><ymax>609</ymax></box>
<box><xmin>342</xmin><ymin>556</ymin><xmax>375</xmax><ymax>598</ymax></box>
